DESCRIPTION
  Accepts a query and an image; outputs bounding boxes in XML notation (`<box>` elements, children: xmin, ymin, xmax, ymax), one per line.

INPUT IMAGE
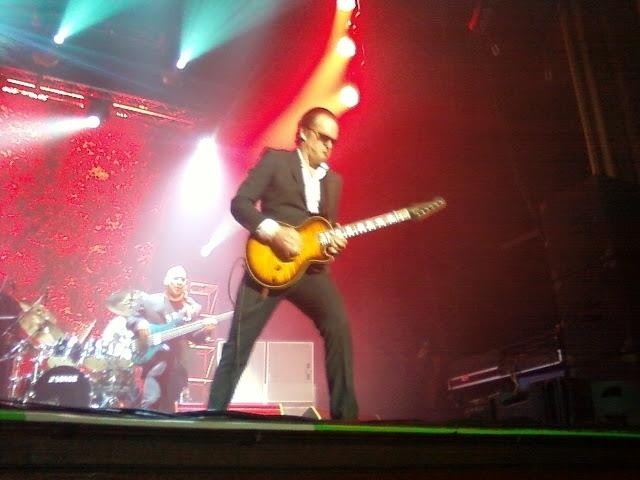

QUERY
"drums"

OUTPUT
<box><xmin>7</xmin><ymin>352</ymin><xmax>48</xmax><ymax>401</ymax></box>
<box><xmin>80</xmin><ymin>337</ymin><xmax>113</xmax><ymax>375</ymax></box>
<box><xmin>32</xmin><ymin>366</ymin><xmax>92</xmax><ymax>409</ymax></box>
<box><xmin>43</xmin><ymin>333</ymin><xmax>82</xmax><ymax>368</ymax></box>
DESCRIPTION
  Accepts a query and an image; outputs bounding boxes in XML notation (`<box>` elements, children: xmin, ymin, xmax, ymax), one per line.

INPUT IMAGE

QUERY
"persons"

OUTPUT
<box><xmin>126</xmin><ymin>265</ymin><xmax>216</xmax><ymax>412</ymax></box>
<box><xmin>205</xmin><ymin>108</ymin><xmax>358</xmax><ymax>422</ymax></box>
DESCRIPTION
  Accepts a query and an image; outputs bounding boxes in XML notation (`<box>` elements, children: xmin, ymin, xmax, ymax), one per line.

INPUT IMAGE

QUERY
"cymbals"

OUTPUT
<box><xmin>14</xmin><ymin>296</ymin><xmax>57</xmax><ymax>324</ymax></box>
<box><xmin>16</xmin><ymin>312</ymin><xmax>64</xmax><ymax>344</ymax></box>
<box><xmin>105</xmin><ymin>290</ymin><xmax>148</xmax><ymax>316</ymax></box>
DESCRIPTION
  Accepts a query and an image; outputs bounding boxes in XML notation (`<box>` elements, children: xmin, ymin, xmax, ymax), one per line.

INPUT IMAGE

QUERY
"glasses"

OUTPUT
<box><xmin>308</xmin><ymin>125</ymin><xmax>338</xmax><ymax>146</ymax></box>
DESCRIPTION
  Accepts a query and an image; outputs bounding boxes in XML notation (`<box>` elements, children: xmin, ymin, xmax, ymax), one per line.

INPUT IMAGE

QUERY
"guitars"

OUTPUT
<box><xmin>129</xmin><ymin>312</ymin><xmax>234</xmax><ymax>365</ymax></box>
<box><xmin>246</xmin><ymin>197</ymin><xmax>446</xmax><ymax>289</ymax></box>
